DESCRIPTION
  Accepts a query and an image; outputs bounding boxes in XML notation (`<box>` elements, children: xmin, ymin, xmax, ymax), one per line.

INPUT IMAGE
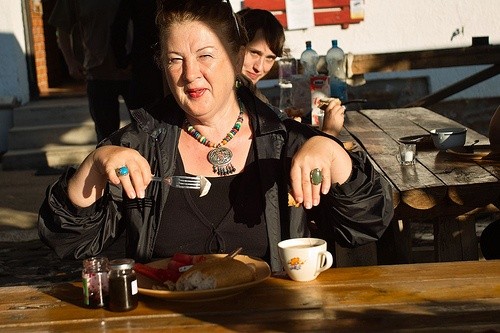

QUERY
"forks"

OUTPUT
<box><xmin>153</xmin><ymin>176</ymin><xmax>201</xmax><ymax>190</ymax></box>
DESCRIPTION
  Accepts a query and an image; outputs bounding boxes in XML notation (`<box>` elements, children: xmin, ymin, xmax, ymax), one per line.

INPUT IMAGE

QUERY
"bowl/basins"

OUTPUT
<box><xmin>429</xmin><ymin>127</ymin><xmax>466</xmax><ymax>151</ymax></box>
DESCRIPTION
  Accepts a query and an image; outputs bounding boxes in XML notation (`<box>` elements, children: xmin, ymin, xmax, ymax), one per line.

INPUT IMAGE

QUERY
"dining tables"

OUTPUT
<box><xmin>334</xmin><ymin>316</ymin><xmax>337</xmax><ymax>319</ymax></box>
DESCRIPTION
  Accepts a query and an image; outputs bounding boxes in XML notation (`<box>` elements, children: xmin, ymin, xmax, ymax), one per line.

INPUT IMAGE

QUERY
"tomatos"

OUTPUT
<box><xmin>132</xmin><ymin>252</ymin><xmax>194</xmax><ymax>281</ymax></box>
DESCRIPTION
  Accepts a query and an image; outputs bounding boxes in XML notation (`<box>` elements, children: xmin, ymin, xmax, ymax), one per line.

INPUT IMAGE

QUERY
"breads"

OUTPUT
<box><xmin>176</xmin><ymin>257</ymin><xmax>255</xmax><ymax>292</ymax></box>
<box><xmin>288</xmin><ymin>189</ymin><xmax>300</xmax><ymax>208</ymax></box>
<box><xmin>318</xmin><ymin>98</ymin><xmax>332</xmax><ymax>111</ymax></box>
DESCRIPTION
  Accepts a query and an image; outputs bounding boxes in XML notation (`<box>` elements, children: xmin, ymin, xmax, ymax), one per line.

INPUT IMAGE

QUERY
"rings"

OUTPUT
<box><xmin>310</xmin><ymin>168</ymin><xmax>323</xmax><ymax>185</ymax></box>
<box><xmin>116</xmin><ymin>167</ymin><xmax>128</xmax><ymax>176</ymax></box>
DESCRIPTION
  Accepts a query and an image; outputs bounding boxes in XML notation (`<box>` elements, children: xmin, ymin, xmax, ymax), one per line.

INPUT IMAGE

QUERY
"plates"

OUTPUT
<box><xmin>398</xmin><ymin>135</ymin><xmax>435</xmax><ymax>152</ymax></box>
<box><xmin>446</xmin><ymin>145</ymin><xmax>495</xmax><ymax>159</ymax></box>
<box><xmin>135</xmin><ymin>253</ymin><xmax>271</xmax><ymax>303</ymax></box>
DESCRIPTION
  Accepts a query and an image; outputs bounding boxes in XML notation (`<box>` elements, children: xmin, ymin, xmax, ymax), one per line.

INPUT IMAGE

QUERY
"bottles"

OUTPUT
<box><xmin>278</xmin><ymin>48</ymin><xmax>297</xmax><ymax>109</ymax></box>
<box><xmin>300</xmin><ymin>42</ymin><xmax>320</xmax><ymax>77</ymax></box>
<box><xmin>82</xmin><ymin>256</ymin><xmax>138</xmax><ymax>311</ymax></box>
<box><xmin>489</xmin><ymin>105</ymin><xmax>500</xmax><ymax>156</ymax></box>
<box><xmin>326</xmin><ymin>39</ymin><xmax>347</xmax><ymax>98</ymax></box>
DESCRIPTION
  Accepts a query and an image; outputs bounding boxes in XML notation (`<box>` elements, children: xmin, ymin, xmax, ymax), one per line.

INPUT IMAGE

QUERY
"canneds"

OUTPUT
<box><xmin>107</xmin><ymin>259</ymin><xmax>139</xmax><ymax>312</ymax></box>
<box><xmin>81</xmin><ymin>256</ymin><xmax>109</xmax><ymax>308</ymax></box>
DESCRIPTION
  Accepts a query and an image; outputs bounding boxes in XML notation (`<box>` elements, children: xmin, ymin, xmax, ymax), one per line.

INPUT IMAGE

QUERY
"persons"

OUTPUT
<box><xmin>480</xmin><ymin>105</ymin><xmax>500</xmax><ymax>260</ymax></box>
<box><xmin>36</xmin><ymin>0</ymin><xmax>394</xmax><ymax>282</ymax></box>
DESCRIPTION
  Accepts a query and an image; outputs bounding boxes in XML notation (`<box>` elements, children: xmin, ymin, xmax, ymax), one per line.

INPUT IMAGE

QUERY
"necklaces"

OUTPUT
<box><xmin>181</xmin><ymin>96</ymin><xmax>244</xmax><ymax>176</ymax></box>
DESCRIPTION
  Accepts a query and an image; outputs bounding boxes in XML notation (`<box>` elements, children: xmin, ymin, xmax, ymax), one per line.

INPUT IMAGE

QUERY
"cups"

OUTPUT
<box><xmin>395</xmin><ymin>143</ymin><xmax>417</xmax><ymax>167</ymax></box>
<box><xmin>278</xmin><ymin>237</ymin><xmax>333</xmax><ymax>282</ymax></box>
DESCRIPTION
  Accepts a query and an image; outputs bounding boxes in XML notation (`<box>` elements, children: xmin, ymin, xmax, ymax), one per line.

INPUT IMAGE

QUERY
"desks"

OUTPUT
<box><xmin>0</xmin><ymin>260</ymin><xmax>500</xmax><ymax>333</ymax></box>
<box><xmin>307</xmin><ymin>104</ymin><xmax>500</xmax><ymax>263</ymax></box>
